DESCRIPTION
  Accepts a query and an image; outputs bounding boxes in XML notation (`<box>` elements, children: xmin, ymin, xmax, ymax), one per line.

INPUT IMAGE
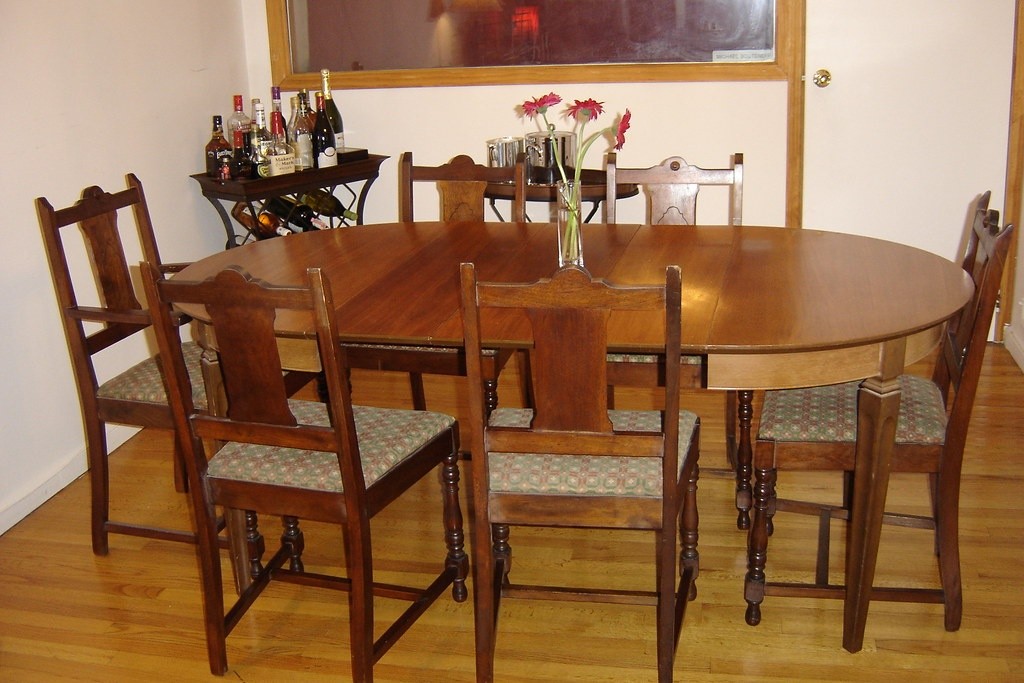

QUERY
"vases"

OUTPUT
<box><xmin>558</xmin><ymin>178</ymin><xmax>581</xmax><ymax>268</ymax></box>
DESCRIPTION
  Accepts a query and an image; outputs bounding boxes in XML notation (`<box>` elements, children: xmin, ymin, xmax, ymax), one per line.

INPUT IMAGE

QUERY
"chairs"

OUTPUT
<box><xmin>739</xmin><ymin>192</ymin><xmax>1015</xmax><ymax>627</ymax></box>
<box><xmin>607</xmin><ymin>154</ymin><xmax>754</xmax><ymax>476</ymax></box>
<box><xmin>140</xmin><ymin>260</ymin><xmax>467</xmax><ymax>683</ymax></box>
<box><xmin>338</xmin><ymin>151</ymin><xmax>529</xmax><ymax>456</ymax></box>
<box><xmin>32</xmin><ymin>171</ymin><xmax>333</xmax><ymax>556</ymax></box>
<box><xmin>459</xmin><ymin>263</ymin><xmax>698</xmax><ymax>683</ymax></box>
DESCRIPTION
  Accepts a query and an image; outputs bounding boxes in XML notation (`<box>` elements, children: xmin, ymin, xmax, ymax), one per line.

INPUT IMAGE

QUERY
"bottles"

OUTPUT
<box><xmin>205</xmin><ymin>86</ymin><xmax>295</xmax><ymax>185</ymax></box>
<box><xmin>313</xmin><ymin>91</ymin><xmax>338</xmax><ymax>169</ymax></box>
<box><xmin>321</xmin><ymin>68</ymin><xmax>344</xmax><ymax>149</ymax></box>
<box><xmin>231</xmin><ymin>196</ymin><xmax>292</xmax><ymax>239</ymax></box>
<box><xmin>286</xmin><ymin>97</ymin><xmax>314</xmax><ymax>171</ymax></box>
<box><xmin>297</xmin><ymin>93</ymin><xmax>314</xmax><ymax>138</ymax></box>
<box><xmin>299</xmin><ymin>89</ymin><xmax>317</xmax><ymax>128</ymax></box>
<box><xmin>297</xmin><ymin>189</ymin><xmax>358</xmax><ymax>221</ymax></box>
<box><xmin>263</xmin><ymin>197</ymin><xmax>331</xmax><ymax>233</ymax></box>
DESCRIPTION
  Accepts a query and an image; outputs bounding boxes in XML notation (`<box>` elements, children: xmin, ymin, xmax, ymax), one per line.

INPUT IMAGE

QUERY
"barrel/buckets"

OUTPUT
<box><xmin>526</xmin><ymin>123</ymin><xmax>580</xmax><ymax>186</ymax></box>
<box><xmin>486</xmin><ymin>135</ymin><xmax>525</xmax><ymax>185</ymax></box>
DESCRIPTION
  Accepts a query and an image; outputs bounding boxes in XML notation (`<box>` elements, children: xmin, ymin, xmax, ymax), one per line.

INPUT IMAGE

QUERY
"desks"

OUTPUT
<box><xmin>453</xmin><ymin>170</ymin><xmax>639</xmax><ymax>224</ymax></box>
<box><xmin>164</xmin><ymin>223</ymin><xmax>973</xmax><ymax>654</ymax></box>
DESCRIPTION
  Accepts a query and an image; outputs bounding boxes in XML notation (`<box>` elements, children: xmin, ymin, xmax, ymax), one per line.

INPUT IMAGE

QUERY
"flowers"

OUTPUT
<box><xmin>523</xmin><ymin>92</ymin><xmax>631</xmax><ymax>265</ymax></box>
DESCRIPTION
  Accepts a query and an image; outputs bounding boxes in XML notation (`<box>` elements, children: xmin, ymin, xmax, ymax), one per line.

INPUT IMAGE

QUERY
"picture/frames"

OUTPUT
<box><xmin>264</xmin><ymin>0</ymin><xmax>790</xmax><ymax>92</ymax></box>
